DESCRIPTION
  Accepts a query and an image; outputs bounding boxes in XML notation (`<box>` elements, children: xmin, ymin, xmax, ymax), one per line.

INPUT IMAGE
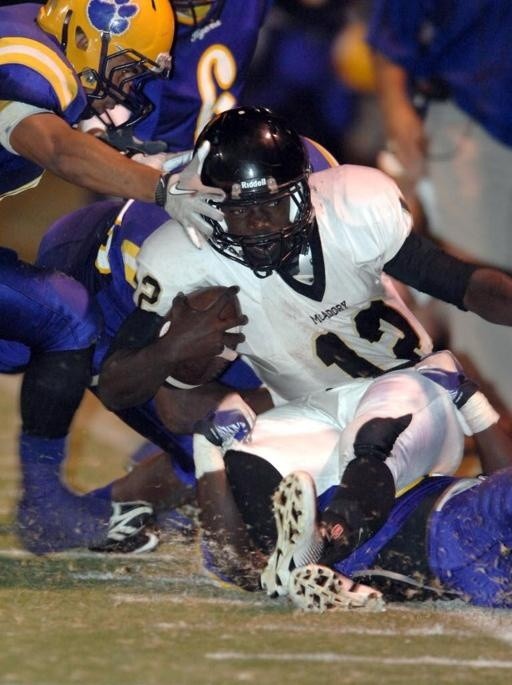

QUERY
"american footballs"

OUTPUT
<box><xmin>154</xmin><ymin>285</ymin><xmax>242</xmax><ymax>391</ymax></box>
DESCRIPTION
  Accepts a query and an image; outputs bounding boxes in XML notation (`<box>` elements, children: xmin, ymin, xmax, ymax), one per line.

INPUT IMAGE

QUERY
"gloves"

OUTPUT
<box><xmin>93</xmin><ymin>125</ymin><xmax>168</xmax><ymax>160</ymax></box>
<box><xmin>153</xmin><ymin>140</ymin><xmax>227</xmax><ymax>250</ymax></box>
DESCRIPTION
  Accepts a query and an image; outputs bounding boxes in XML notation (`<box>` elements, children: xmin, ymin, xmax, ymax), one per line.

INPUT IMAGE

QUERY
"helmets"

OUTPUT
<box><xmin>193</xmin><ymin>105</ymin><xmax>315</xmax><ymax>273</ymax></box>
<box><xmin>35</xmin><ymin>1</ymin><xmax>225</xmax><ymax>134</ymax></box>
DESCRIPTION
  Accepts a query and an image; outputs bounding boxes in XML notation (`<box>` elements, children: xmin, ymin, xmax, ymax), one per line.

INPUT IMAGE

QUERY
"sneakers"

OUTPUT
<box><xmin>157</xmin><ymin>508</ymin><xmax>198</xmax><ymax>542</ymax></box>
<box><xmin>288</xmin><ymin>564</ymin><xmax>387</xmax><ymax>612</ymax></box>
<box><xmin>18</xmin><ymin>498</ymin><xmax>154</xmax><ymax>554</ymax></box>
<box><xmin>259</xmin><ymin>471</ymin><xmax>325</xmax><ymax>600</ymax></box>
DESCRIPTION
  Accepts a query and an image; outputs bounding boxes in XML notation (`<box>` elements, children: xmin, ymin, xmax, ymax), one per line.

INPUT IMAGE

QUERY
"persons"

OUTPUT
<box><xmin>0</xmin><ymin>0</ymin><xmax>512</xmax><ymax>613</ymax></box>
